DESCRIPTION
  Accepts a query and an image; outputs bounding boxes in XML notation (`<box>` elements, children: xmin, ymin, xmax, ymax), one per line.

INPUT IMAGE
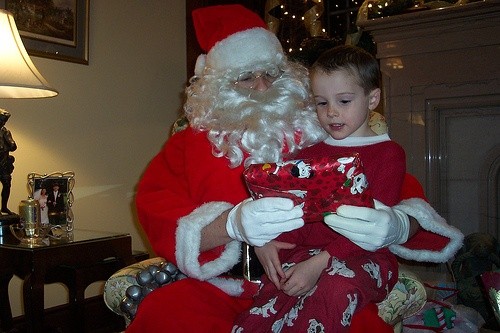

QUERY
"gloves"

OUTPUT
<box><xmin>226</xmin><ymin>196</ymin><xmax>306</xmax><ymax>248</ymax></box>
<box><xmin>323</xmin><ymin>198</ymin><xmax>409</xmax><ymax>252</ymax></box>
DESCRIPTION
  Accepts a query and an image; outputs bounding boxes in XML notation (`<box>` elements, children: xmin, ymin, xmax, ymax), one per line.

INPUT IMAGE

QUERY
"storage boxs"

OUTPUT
<box><xmin>243</xmin><ymin>151</ymin><xmax>375</xmax><ymax>222</ymax></box>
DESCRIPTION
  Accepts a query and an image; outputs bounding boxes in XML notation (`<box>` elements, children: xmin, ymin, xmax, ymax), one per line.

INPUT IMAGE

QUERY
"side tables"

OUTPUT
<box><xmin>0</xmin><ymin>229</ymin><xmax>132</xmax><ymax>332</ymax></box>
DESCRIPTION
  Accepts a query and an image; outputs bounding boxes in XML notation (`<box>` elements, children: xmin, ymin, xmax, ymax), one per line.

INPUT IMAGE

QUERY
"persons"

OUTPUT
<box><xmin>232</xmin><ymin>43</ymin><xmax>406</xmax><ymax>333</ymax></box>
<box><xmin>46</xmin><ymin>184</ymin><xmax>65</xmax><ymax>225</ymax></box>
<box><xmin>36</xmin><ymin>187</ymin><xmax>51</xmax><ymax>224</ymax></box>
<box><xmin>126</xmin><ymin>5</ymin><xmax>464</xmax><ymax>333</ymax></box>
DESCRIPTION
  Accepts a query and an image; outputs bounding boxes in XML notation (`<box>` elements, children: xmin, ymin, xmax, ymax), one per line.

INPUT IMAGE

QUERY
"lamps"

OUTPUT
<box><xmin>1</xmin><ymin>9</ymin><xmax>59</xmax><ymax>99</ymax></box>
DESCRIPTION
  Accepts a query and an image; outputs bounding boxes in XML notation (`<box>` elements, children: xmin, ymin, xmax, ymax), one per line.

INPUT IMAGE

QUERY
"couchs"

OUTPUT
<box><xmin>103</xmin><ymin>256</ymin><xmax>427</xmax><ymax>332</ymax></box>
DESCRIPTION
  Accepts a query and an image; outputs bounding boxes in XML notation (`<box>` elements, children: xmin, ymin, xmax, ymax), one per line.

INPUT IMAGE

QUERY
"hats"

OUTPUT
<box><xmin>188</xmin><ymin>3</ymin><xmax>286</xmax><ymax>77</ymax></box>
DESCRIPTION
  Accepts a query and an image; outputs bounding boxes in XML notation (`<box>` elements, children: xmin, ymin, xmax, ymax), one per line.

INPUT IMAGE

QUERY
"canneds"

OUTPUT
<box><xmin>19</xmin><ymin>199</ymin><xmax>41</xmax><ymax>237</ymax></box>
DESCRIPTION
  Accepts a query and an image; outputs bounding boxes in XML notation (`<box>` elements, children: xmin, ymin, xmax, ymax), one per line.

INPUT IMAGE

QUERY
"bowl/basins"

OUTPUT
<box><xmin>10</xmin><ymin>223</ymin><xmax>52</xmax><ymax>244</ymax></box>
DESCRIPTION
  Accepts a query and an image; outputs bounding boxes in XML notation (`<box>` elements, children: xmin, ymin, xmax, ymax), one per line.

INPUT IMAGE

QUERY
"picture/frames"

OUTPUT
<box><xmin>0</xmin><ymin>0</ymin><xmax>90</xmax><ymax>65</ymax></box>
<box><xmin>27</xmin><ymin>170</ymin><xmax>75</xmax><ymax>231</ymax></box>
